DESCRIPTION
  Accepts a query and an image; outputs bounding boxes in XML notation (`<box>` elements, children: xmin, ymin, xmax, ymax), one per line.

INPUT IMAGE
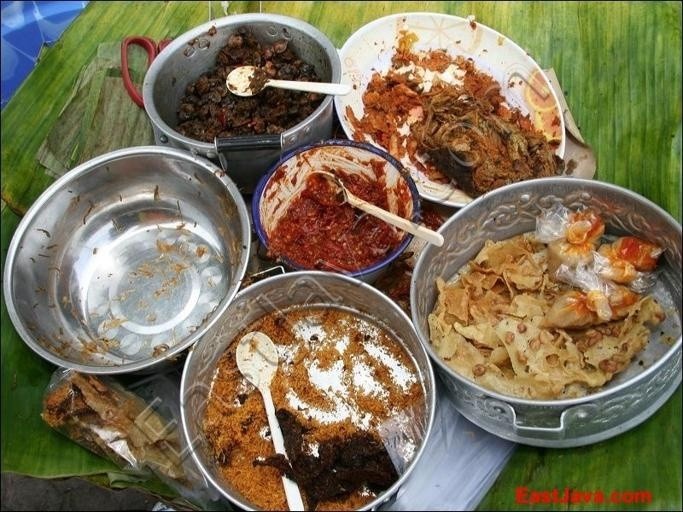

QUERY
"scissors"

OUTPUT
<box><xmin>120</xmin><ymin>35</ymin><xmax>180</xmax><ymax>147</ymax></box>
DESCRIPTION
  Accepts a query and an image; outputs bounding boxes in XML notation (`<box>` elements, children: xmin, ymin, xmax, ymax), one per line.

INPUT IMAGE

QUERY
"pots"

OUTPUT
<box><xmin>174</xmin><ymin>269</ymin><xmax>433</xmax><ymax>512</ymax></box>
<box><xmin>138</xmin><ymin>1</ymin><xmax>340</xmax><ymax>201</ymax></box>
<box><xmin>405</xmin><ymin>177</ymin><xmax>682</xmax><ymax>438</ymax></box>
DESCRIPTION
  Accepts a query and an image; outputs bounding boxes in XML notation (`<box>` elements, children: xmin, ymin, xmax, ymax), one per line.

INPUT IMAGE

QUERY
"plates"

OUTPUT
<box><xmin>331</xmin><ymin>13</ymin><xmax>566</xmax><ymax>211</ymax></box>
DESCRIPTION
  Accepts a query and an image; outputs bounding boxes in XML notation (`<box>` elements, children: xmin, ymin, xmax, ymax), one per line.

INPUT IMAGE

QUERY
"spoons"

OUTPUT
<box><xmin>223</xmin><ymin>63</ymin><xmax>355</xmax><ymax>103</ymax></box>
<box><xmin>235</xmin><ymin>331</ymin><xmax>307</xmax><ymax>511</ymax></box>
<box><xmin>305</xmin><ymin>173</ymin><xmax>446</xmax><ymax>247</ymax></box>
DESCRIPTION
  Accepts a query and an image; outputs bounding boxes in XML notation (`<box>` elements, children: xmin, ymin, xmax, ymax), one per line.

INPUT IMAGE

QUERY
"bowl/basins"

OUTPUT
<box><xmin>251</xmin><ymin>141</ymin><xmax>422</xmax><ymax>284</ymax></box>
<box><xmin>4</xmin><ymin>149</ymin><xmax>255</xmax><ymax>381</ymax></box>
